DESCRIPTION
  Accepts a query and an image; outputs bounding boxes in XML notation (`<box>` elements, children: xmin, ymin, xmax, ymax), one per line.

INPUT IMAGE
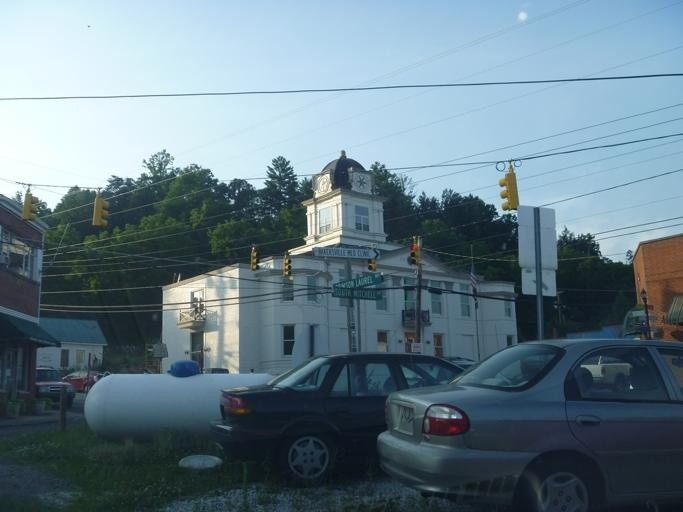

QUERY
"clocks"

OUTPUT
<box><xmin>316</xmin><ymin>174</ymin><xmax>332</xmax><ymax>197</ymax></box>
<box><xmin>352</xmin><ymin>173</ymin><xmax>373</xmax><ymax>195</ymax></box>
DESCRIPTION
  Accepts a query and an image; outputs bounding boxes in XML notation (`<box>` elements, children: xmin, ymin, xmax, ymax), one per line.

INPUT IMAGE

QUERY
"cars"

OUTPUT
<box><xmin>211</xmin><ymin>350</ymin><xmax>470</xmax><ymax>489</ymax></box>
<box><xmin>374</xmin><ymin>337</ymin><xmax>683</xmax><ymax>512</ymax></box>
<box><xmin>35</xmin><ymin>363</ymin><xmax>99</xmax><ymax>408</ymax></box>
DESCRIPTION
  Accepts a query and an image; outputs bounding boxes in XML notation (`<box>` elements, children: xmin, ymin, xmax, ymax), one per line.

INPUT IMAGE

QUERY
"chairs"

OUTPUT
<box><xmin>498</xmin><ymin>358</ymin><xmax>674</xmax><ymax>399</ymax></box>
<box><xmin>347</xmin><ymin>376</ymin><xmax>397</xmax><ymax>395</ymax></box>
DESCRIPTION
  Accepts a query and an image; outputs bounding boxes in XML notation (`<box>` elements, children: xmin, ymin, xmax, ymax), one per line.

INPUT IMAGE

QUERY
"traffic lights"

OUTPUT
<box><xmin>410</xmin><ymin>237</ymin><xmax>421</xmax><ymax>266</ymax></box>
<box><xmin>499</xmin><ymin>166</ymin><xmax>519</xmax><ymax>213</ymax></box>
<box><xmin>250</xmin><ymin>247</ymin><xmax>259</xmax><ymax>273</ymax></box>
<box><xmin>367</xmin><ymin>258</ymin><xmax>375</xmax><ymax>272</ymax></box>
<box><xmin>91</xmin><ymin>196</ymin><xmax>109</xmax><ymax>227</ymax></box>
<box><xmin>22</xmin><ymin>192</ymin><xmax>39</xmax><ymax>221</ymax></box>
<box><xmin>283</xmin><ymin>254</ymin><xmax>292</xmax><ymax>279</ymax></box>
